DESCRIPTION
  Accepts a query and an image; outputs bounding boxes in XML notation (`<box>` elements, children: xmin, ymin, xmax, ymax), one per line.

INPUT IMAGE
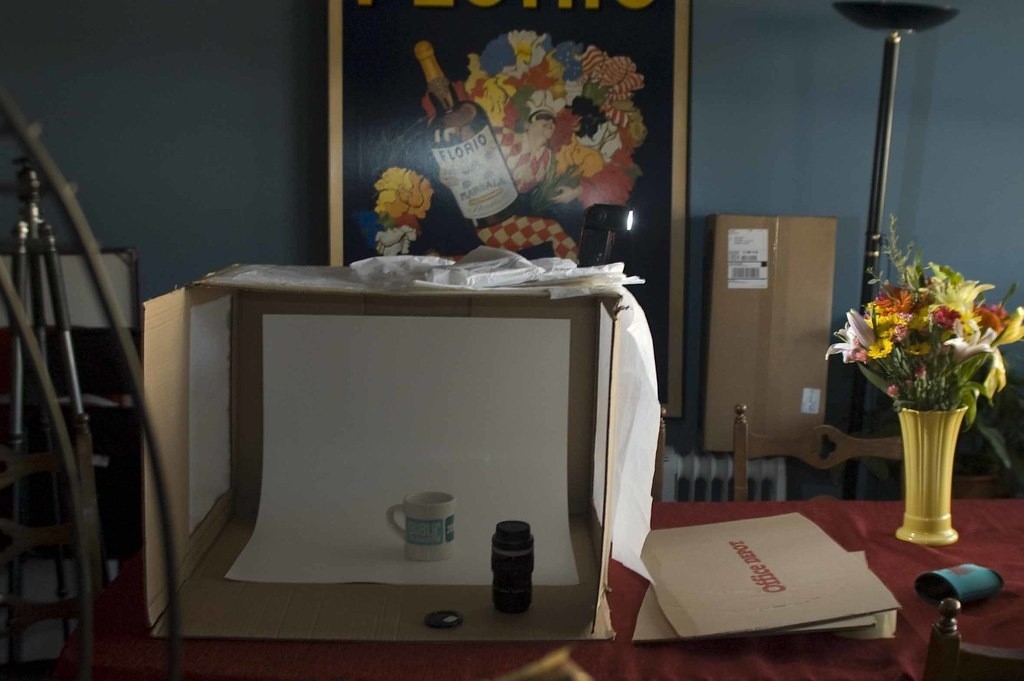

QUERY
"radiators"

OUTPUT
<box><xmin>660</xmin><ymin>446</ymin><xmax>787</xmax><ymax>502</ymax></box>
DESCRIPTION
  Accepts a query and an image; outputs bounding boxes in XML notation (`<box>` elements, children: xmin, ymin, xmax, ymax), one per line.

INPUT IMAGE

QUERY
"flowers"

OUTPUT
<box><xmin>823</xmin><ymin>212</ymin><xmax>1024</xmax><ymax>434</ymax></box>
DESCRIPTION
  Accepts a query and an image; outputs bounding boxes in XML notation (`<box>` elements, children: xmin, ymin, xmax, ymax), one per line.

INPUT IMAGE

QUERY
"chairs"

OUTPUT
<box><xmin>729</xmin><ymin>405</ymin><xmax>997</xmax><ymax>505</ymax></box>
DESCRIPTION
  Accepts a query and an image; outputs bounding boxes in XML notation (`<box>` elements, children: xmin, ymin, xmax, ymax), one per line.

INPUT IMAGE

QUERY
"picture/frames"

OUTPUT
<box><xmin>327</xmin><ymin>0</ymin><xmax>692</xmax><ymax>421</ymax></box>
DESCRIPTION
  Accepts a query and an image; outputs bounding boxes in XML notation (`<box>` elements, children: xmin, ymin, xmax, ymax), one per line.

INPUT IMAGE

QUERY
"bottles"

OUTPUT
<box><xmin>490</xmin><ymin>520</ymin><xmax>537</xmax><ymax>614</ymax></box>
<box><xmin>414</xmin><ymin>42</ymin><xmax>522</xmax><ymax>228</ymax></box>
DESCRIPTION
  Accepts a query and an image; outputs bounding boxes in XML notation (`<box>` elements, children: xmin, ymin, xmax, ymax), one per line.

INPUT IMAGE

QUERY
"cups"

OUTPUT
<box><xmin>388</xmin><ymin>490</ymin><xmax>455</xmax><ymax>562</ymax></box>
<box><xmin>912</xmin><ymin>563</ymin><xmax>1004</xmax><ymax>609</ymax></box>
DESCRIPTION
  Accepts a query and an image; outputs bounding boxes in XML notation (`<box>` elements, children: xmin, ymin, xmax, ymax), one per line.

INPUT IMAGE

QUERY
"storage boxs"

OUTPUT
<box><xmin>139</xmin><ymin>265</ymin><xmax>630</xmax><ymax>641</ymax></box>
<box><xmin>703</xmin><ymin>213</ymin><xmax>838</xmax><ymax>460</ymax></box>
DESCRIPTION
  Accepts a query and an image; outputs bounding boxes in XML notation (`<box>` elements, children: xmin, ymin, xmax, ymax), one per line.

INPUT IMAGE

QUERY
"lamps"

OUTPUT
<box><xmin>577</xmin><ymin>203</ymin><xmax>636</xmax><ymax>268</ymax></box>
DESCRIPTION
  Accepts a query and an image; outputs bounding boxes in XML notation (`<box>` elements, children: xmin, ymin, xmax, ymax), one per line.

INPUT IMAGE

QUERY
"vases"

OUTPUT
<box><xmin>895</xmin><ymin>406</ymin><xmax>970</xmax><ymax>546</ymax></box>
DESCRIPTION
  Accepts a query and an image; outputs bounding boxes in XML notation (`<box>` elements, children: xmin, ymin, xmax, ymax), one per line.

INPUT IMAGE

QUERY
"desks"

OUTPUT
<box><xmin>52</xmin><ymin>500</ymin><xmax>1024</xmax><ymax>681</ymax></box>
